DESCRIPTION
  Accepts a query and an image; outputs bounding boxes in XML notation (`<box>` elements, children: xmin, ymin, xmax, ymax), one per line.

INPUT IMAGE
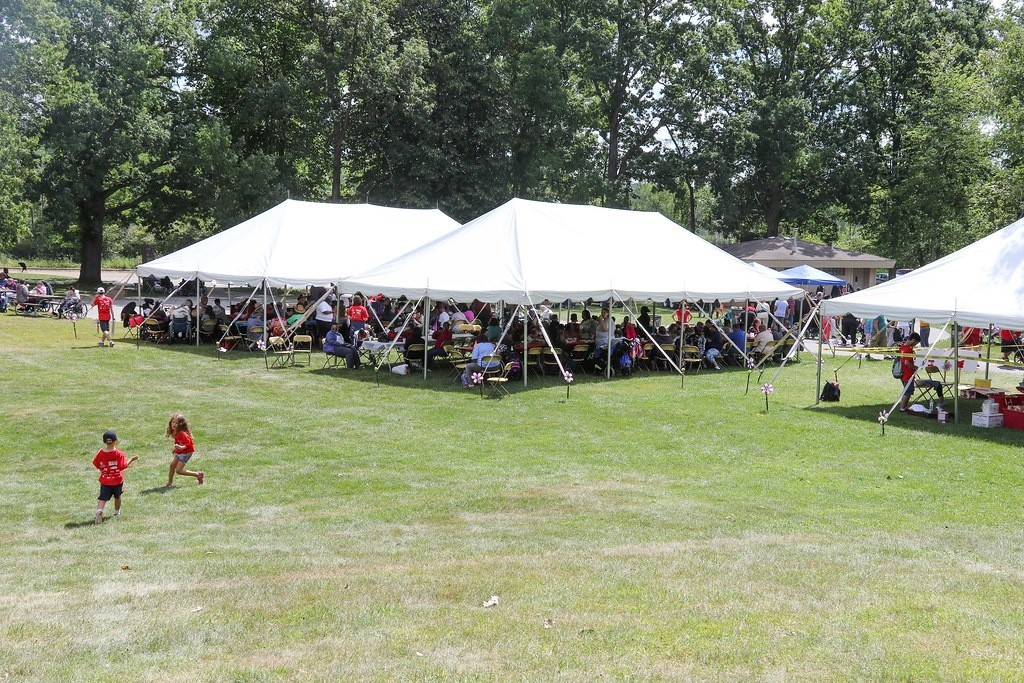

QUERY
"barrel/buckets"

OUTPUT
<box><xmin>975</xmin><ymin>379</ymin><xmax>992</xmax><ymax>389</ymax></box>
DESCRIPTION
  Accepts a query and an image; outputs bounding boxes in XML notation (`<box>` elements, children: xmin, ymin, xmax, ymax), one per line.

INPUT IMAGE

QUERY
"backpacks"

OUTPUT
<box><xmin>892</xmin><ymin>356</ymin><xmax>902</xmax><ymax>379</ymax></box>
<box><xmin>819</xmin><ymin>380</ymin><xmax>840</xmax><ymax>402</ymax></box>
<box><xmin>508</xmin><ymin>357</ymin><xmax>522</xmax><ymax>380</ymax></box>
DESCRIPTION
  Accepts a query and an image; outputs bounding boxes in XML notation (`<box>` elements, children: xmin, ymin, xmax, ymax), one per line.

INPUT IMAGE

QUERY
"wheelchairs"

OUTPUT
<box><xmin>1013</xmin><ymin>340</ymin><xmax>1024</xmax><ymax>364</ymax></box>
<box><xmin>54</xmin><ymin>290</ymin><xmax>88</xmax><ymax>319</ymax></box>
<box><xmin>15</xmin><ymin>280</ymin><xmax>53</xmax><ymax>317</ymax></box>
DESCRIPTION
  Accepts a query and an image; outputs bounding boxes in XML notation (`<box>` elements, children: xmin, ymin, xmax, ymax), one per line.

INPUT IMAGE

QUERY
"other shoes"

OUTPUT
<box><xmin>110</xmin><ymin>342</ymin><xmax>114</xmax><ymax>347</ymax></box>
<box><xmin>197</xmin><ymin>471</ymin><xmax>203</xmax><ymax>484</ymax></box>
<box><xmin>900</xmin><ymin>408</ymin><xmax>908</xmax><ymax>414</ymax></box>
<box><xmin>114</xmin><ymin>512</ymin><xmax>121</xmax><ymax>516</ymax></box>
<box><xmin>95</xmin><ymin>510</ymin><xmax>103</xmax><ymax>524</ymax></box>
<box><xmin>97</xmin><ymin>342</ymin><xmax>104</xmax><ymax>345</ymax></box>
<box><xmin>163</xmin><ymin>482</ymin><xmax>173</xmax><ymax>487</ymax></box>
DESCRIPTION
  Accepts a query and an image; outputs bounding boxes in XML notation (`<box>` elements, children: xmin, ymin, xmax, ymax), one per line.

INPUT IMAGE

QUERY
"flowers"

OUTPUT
<box><xmin>762</xmin><ymin>384</ymin><xmax>774</xmax><ymax>411</ymax></box>
<box><xmin>563</xmin><ymin>370</ymin><xmax>574</xmax><ymax>398</ymax></box>
<box><xmin>256</xmin><ymin>339</ymin><xmax>268</xmax><ymax>370</ymax></box>
<box><xmin>67</xmin><ymin>314</ymin><xmax>77</xmax><ymax>338</ymax></box>
<box><xmin>471</xmin><ymin>373</ymin><xmax>483</xmax><ymax>396</ymax></box>
<box><xmin>878</xmin><ymin>410</ymin><xmax>889</xmax><ymax>434</ymax></box>
<box><xmin>942</xmin><ymin>362</ymin><xmax>952</xmax><ymax>379</ymax></box>
<box><xmin>855</xmin><ymin>353</ymin><xmax>863</xmax><ymax>369</ymax></box>
<box><xmin>94</xmin><ymin>318</ymin><xmax>100</xmax><ymax>334</ymax></box>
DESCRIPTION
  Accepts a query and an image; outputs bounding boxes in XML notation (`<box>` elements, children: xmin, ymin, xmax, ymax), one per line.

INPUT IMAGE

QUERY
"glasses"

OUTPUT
<box><xmin>332</xmin><ymin>328</ymin><xmax>338</xmax><ymax>330</ymax></box>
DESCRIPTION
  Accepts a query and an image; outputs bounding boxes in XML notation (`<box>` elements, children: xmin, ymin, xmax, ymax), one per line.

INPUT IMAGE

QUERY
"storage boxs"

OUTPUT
<box><xmin>959</xmin><ymin>380</ymin><xmax>1024</xmax><ymax>430</ymax></box>
<box><xmin>938</xmin><ymin>411</ymin><xmax>948</xmax><ymax>423</ymax></box>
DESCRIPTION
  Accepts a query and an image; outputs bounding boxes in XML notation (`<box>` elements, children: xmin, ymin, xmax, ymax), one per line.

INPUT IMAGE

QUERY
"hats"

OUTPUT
<box><xmin>634</xmin><ymin>338</ymin><xmax>641</xmax><ymax>344</ymax></box>
<box><xmin>295</xmin><ymin>304</ymin><xmax>306</xmax><ymax>313</ymax></box>
<box><xmin>97</xmin><ymin>287</ymin><xmax>105</xmax><ymax>293</ymax></box>
<box><xmin>103</xmin><ymin>430</ymin><xmax>117</xmax><ymax>443</ymax></box>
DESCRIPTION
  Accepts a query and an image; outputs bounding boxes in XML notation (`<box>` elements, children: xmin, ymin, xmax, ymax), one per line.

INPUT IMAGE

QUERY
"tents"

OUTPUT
<box><xmin>136</xmin><ymin>198</ymin><xmax>464</xmax><ymax>357</ymax></box>
<box><xmin>816</xmin><ymin>217</ymin><xmax>1024</xmax><ymax>405</ymax></box>
<box><xmin>335</xmin><ymin>198</ymin><xmax>805</xmax><ymax>388</ymax></box>
<box><xmin>746</xmin><ymin>263</ymin><xmax>848</xmax><ymax>298</ymax></box>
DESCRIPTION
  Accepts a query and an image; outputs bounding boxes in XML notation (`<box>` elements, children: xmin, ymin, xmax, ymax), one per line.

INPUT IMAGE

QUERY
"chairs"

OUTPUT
<box><xmin>911</xmin><ymin>365</ymin><xmax>956</xmax><ymax>408</ymax></box>
<box><xmin>122</xmin><ymin>304</ymin><xmax>797</xmax><ymax>397</ymax></box>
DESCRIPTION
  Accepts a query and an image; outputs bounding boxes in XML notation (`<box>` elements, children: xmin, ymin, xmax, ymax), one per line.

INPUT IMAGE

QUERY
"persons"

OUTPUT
<box><xmin>91</xmin><ymin>287</ymin><xmax>116</xmax><ymax>346</ymax></box>
<box><xmin>1000</xmin><ymin>329</ymin><xmax>1021</xmax><ymax>362</ymax></box>
<box><xmin>161</xmin><ymin>415</ymin><xmax>204</xmax><ymax>487</ymax></box>
<box><xmin>142</xmin><ymin>274</ymin><xmax>913</xmax><ymax>387</ymax></box>
<box><xmin>92</xmin><ymin>430</ymin><xmax>140</xmax><ymax>524</ymax></box>
<box><xmin>899</xmin><ymin>333</ymin><xmax>921</xmax><ymax>411</ymax></box>
<box><xmin>0</xmin><ymin>268</ymin><xmax>80</xmax><ymax>319</ymax></box>
<box><xmin>919</xmin><ymin>322</ymin><xmax>931</xmax><ymax>348</ymax></box>
<box><xmin>961</xmin><ymin>327</ymin><xmax>983</xmax><ymax>355</ymax></box>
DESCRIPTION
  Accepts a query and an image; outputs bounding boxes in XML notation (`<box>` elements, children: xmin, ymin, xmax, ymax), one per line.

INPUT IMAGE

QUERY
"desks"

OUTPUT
<box><xmin>29</xmin><ymin>294</ymin><xmax>64</xmax><ymax>315</ymax></box>
<box><xmin>360</xmin><ymin>334</ymin><xmax>474</xmax><ymax>366</ymax></box>
<box><xmin>0</xmin><ymin>289</ymin><xmax>15</xmax><ymax>309</ymax></box>
<box><xmin>234</xmin><ymin>320</ymin><xmax>316</xmax><ymax>334</ymax></box>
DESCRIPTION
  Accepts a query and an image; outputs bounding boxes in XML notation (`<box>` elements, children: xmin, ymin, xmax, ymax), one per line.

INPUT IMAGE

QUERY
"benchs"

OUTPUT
<box><xmin>11</xmin><ymin>301</ymin><xmax>61</xmax><ymax>318</ymax></box>
